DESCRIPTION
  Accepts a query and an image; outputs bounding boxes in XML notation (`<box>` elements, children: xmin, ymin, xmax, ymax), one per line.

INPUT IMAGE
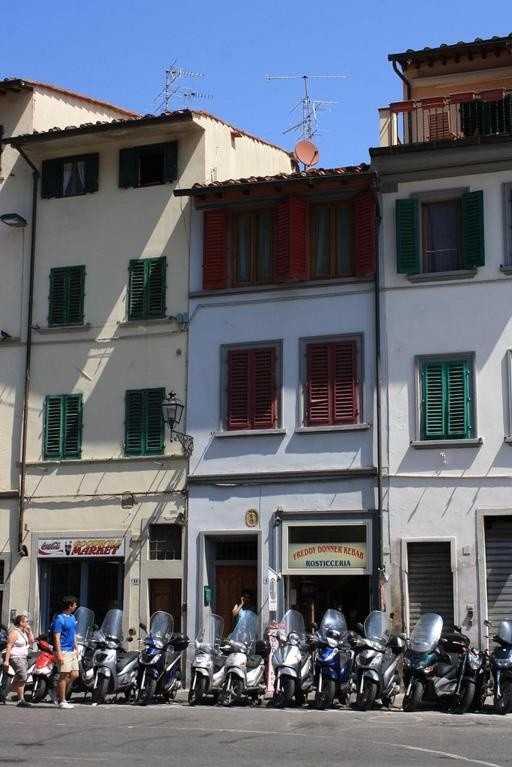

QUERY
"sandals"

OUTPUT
<box><xmin>17</xmin><ymin>699</ymin><xmax>31</xmax><ymax>707</ymax></box>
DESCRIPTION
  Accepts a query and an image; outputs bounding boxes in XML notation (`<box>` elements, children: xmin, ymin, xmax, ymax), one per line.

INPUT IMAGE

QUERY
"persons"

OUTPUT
<box><xmin>0</xmin><ymin>614</ymin><xmax>35</xmax><ymax>707</ymax></box>
<box><xmin>232</xmin><ymin>589</ymin><xmax>257</xmax><ymax>629</ymax></box>
<box><xmin>47</xmin><ymin>595</ymin><xmax>80</xmax><ymax>708</ymax></box>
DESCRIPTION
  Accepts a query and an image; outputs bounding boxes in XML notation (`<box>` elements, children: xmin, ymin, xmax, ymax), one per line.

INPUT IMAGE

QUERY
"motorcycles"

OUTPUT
<box><xmin>483</xmin><ymin>616</ymin><xmax>511</xmax><ymax>715</ymax></box>
<box><xmin>0</xmin><ymin>606</ymin><xmax>188</xmax><ymax>705</ymax></box>
<box><xmin>188</xmin><ymin>613</ymin><xmax>233</xmax><ymax>706</ymax></box>
<box><xmin>219</xmin><ymin>610</ymin><xmax>272</xmax><ymax>706</ymax></box>
<box><xmin>267</xmin><ymin>608</ymin><xmax>492</xmax><ymax>713</ymax></box>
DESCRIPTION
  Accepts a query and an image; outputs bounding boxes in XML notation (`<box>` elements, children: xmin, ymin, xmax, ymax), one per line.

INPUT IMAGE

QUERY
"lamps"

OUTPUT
<box><xmin>163</xmin><ymin>391</ymin><xmax>194</xmax><ymax>454</ymax></box>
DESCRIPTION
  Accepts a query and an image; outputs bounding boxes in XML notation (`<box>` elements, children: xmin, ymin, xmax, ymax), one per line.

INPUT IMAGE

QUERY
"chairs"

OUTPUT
<box><xmin>429</xmin><ymin>112</ymin><xmax>462</xmax><ymax>140</ymax></box>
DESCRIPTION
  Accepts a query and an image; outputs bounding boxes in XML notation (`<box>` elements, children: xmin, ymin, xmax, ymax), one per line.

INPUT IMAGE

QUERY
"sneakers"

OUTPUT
<box><xmin>48</xmin><ymin>689</ymin><xmax>74</xmax><ymax>709</ymax></box>
